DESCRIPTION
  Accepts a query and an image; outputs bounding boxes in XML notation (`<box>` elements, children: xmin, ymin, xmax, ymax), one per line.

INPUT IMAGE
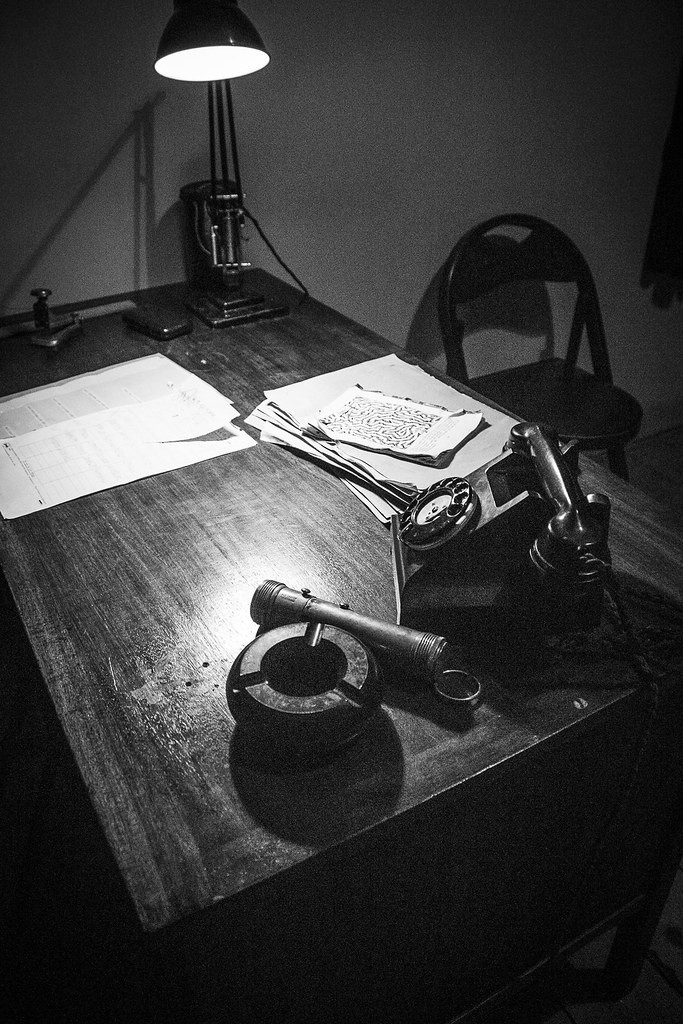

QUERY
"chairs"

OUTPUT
<box><xmin>435</xmin><ymin>212</ymin><xmax>643</xmax><ymax>482</ymax></box>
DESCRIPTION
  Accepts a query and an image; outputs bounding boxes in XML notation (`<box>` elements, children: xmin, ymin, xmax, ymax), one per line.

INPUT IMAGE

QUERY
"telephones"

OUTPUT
<box><xmin>390</xmin><ymin>422</ymin><xmax>614</xmax><ymax>638</ymax></box>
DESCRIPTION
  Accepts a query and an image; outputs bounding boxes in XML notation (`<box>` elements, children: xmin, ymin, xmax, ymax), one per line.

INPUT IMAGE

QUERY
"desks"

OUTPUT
<box><xmin>0</xmin><ymin>267</ymin><xmax>683</xmax><ymax>1024</ymax></box>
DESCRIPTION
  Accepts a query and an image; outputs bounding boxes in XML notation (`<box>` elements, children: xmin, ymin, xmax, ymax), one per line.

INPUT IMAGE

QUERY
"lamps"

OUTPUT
<box><xmin>153</xmin><ymin>0</ymin><xmax>293</xmax><ymax>329</ymax></box>
